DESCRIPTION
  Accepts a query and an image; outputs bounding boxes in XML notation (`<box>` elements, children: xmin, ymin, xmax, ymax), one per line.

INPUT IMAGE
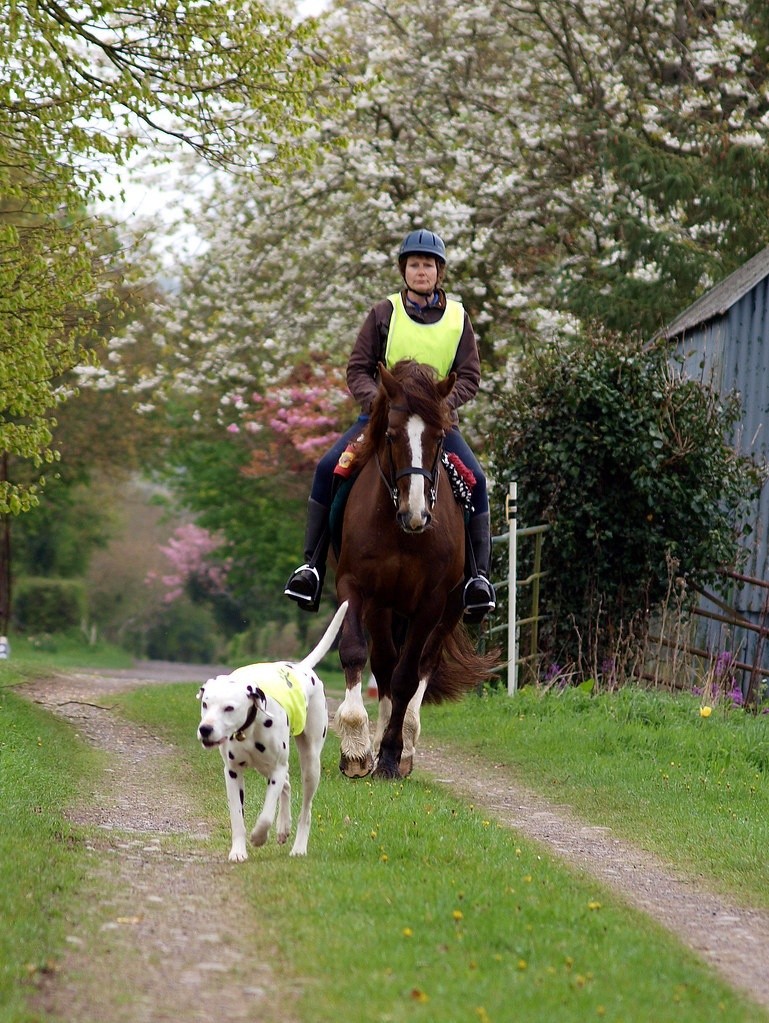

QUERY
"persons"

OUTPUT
<box><xmin>288</xmin><ymin>229</ymin><xmax>492</xmax><ymax>626</ymax></box>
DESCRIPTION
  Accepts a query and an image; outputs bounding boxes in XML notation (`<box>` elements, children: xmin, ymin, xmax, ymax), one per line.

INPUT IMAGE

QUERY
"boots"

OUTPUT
<box><xmin>288</xmin><ymin>497</ymin><xmax>332</xmax><ymax>612</ymax></box>
<box><xmin>462</xmin><ymin>512</ymin><xmax>491</xmax><ymax>624</ymax></box>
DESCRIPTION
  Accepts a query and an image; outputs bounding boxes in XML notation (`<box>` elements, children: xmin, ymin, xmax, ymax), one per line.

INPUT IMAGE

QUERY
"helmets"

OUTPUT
<box><xmin>398</xmin><ymin>229</ymin><xmax>447</xmax><ymax>267</ymax></box>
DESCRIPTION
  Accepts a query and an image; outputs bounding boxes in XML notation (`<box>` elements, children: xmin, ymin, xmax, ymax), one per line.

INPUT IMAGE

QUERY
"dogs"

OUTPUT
<box><xmin>195</xmin><ymin>597</ymin><xmax>351</xmax><ymax>863</ymax></box>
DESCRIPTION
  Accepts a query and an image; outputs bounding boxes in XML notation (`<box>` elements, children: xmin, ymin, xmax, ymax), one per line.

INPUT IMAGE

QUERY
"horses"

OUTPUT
<box><xmin>325</xmin><ymin>356</ymin><xmax>513</xmax><ymax>783</ymax></box>
